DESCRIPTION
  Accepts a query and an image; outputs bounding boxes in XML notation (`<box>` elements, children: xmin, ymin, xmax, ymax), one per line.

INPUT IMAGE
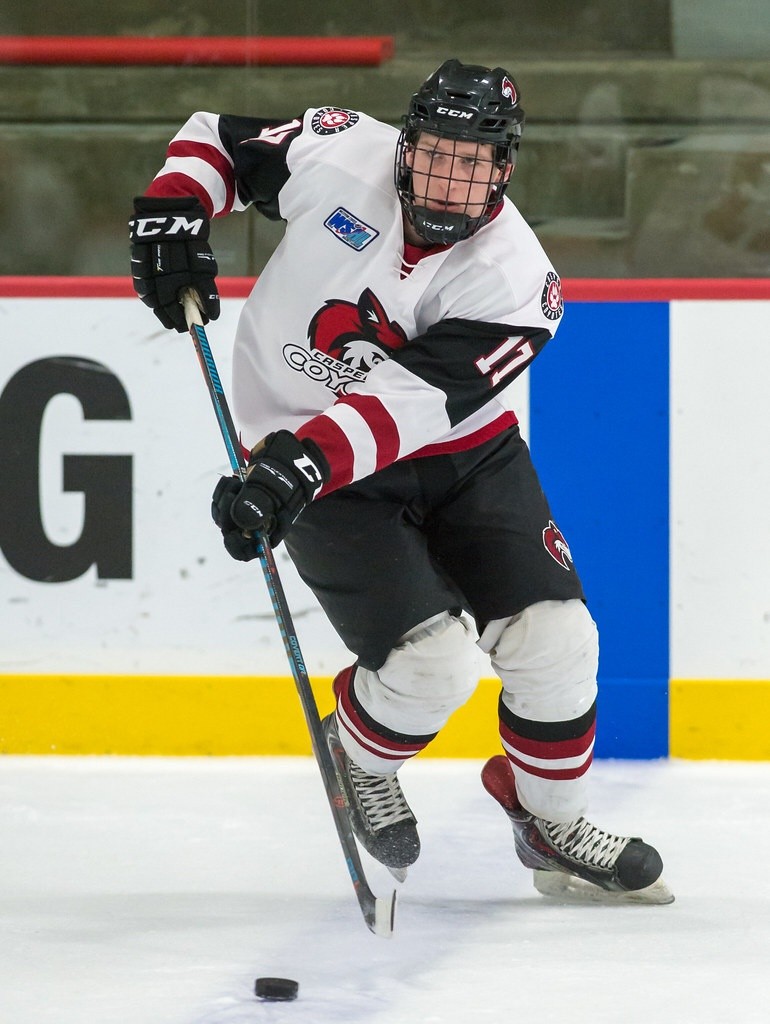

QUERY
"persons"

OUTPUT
<box><xmin>129</xmin><ymin>57</ymin><xmax>675</xmax><ymax>905</ymax></box>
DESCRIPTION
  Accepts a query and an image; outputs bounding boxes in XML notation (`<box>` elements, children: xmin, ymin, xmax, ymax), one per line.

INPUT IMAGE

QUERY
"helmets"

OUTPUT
<box><xmin>393</xmin><ymin>59</ymin><xmax>525</xmax><ymax>243</ymax></box>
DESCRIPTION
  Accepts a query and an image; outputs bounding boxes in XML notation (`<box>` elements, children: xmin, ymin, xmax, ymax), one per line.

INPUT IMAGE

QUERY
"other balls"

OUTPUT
<box><xmin>254</xmin><ymin>977</ymin><xmax>299</xmax><ymax>1002</ymax></box>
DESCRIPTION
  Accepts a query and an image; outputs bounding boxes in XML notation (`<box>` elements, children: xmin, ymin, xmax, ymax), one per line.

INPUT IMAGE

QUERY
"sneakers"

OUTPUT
<box><xmin>480</xmin><ymin>754</ymin><xmax>664</xmax><ymax>893</ymax></box>
<box><xmin>316</xmin><ymin>667</ymin><xmax>421</xmax><ymax>869</ymax></box>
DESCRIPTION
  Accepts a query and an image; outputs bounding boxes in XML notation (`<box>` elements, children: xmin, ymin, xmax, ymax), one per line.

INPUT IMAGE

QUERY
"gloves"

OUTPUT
<box><xmin>128</xmin><ymin>196</ymin><xmax>220</xmax><ymax>334</ymax></box>
<box><xmin>211</xmin><ymin>428</ymin><xmax>330</xmax><ymax>562</ymax></box>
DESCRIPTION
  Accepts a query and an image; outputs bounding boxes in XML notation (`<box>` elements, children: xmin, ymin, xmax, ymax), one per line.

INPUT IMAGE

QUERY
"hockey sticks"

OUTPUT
<box><xmin>180</xmin><ymin>285</ymin><xmax>399</xmax><ymax>940</ymax></box>
